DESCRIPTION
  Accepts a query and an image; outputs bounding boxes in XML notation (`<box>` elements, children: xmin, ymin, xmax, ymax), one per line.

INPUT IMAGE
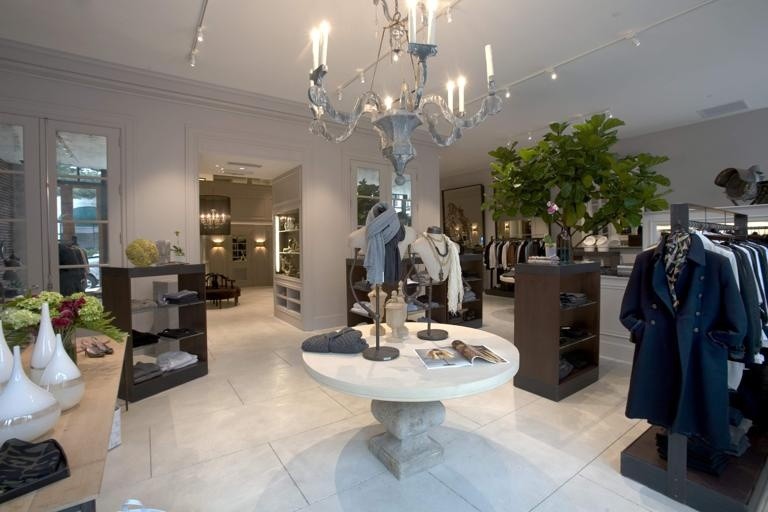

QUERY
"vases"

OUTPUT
<box><xmin>60</xmin><ymin>328</ymin><xmax>77</xmax><ymax>364</ymax></box>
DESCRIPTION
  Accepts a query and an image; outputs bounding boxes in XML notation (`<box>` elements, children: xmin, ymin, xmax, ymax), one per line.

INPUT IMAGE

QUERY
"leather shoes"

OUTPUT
<box><xmin>90</xmin><ymin>337</ymin><xmax>113</xmax><ymax>354</ymax></box>
<box><xmin>80</xmin><ymin>339</ymin><xmax>104</xmax><ymax>358</ymax></box>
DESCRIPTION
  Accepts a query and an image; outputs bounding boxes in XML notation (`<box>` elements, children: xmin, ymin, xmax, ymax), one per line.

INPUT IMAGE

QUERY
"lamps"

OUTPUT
<box><xmin>308</xmin><ymin>0</ymin><xmax>504</xmax><ymax>187</ymax></box>
<box><xmin>332</xmin><ymin>0</ymin><xmax>462</xmax><ymax>102</ymax></box>
<box><xmin>200</xmin><ymin>200</ymin><xmax>227</xmax><ymax>230</ymax></box>
<box><xmin>185</xmin><ymin>0</ymin><xmax>211</xmax><ymax>71</ymax></box>
<box><xmin>442</xmin><ymin>0</ymin><xmax>715</xmax><ymax>108</ymax></box>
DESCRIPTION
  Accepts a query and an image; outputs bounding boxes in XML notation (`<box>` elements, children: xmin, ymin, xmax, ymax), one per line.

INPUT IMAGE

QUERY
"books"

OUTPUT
<box><xmin>414</xmin><ymin>339</ymin><xmax>508</xmax><ymax>370</ymax></box>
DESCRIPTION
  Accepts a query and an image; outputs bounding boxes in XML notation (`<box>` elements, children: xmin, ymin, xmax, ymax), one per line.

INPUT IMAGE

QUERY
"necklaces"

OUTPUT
<box><xmin>419</xmin><ymin>230</ymin><xmax>452</xmax><ymax>281</ymax></box>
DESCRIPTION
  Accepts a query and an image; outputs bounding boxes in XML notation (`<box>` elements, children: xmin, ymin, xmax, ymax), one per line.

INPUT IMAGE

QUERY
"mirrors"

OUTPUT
<box><xmin>201</xmin><ymin>194</ymin><xmax>232</xmax><ymax>235</ymax></box>
<box><xmin>442</xmin><ymin>184</ymin><xmax>486</xmax><ymax>254</ymax></box>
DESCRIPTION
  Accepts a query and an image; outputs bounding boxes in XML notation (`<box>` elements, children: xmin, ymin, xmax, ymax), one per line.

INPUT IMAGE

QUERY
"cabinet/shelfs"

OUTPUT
<box><xmin>271</xmin><ymin>165</ymin><xmax>306</xmax><ymax>332</ymax></box>
<box><xmin>447</xmin><ymin>254</ymin><xmax>483</xmax><ymax>328</ymax></box>
<box><xmin>514</xmin><ymin>260</ymin><xmax>601</xmax><ymax>401</ymax></box>
<box><xmin>100</xmin><ymin>263</ymin><xmax>208</xmax><ymax>403</ymax></box>
<box><xmin>345</xmin><ymin>257</ymin><xmax>446</xmax><ymax>327</ymax></box>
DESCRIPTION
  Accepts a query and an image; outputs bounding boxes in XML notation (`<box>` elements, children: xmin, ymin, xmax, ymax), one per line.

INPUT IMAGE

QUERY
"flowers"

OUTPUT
<box><xmin>1</xmin><ymin>289</ymin><xmax>131</xmax><ymax>362</ymax></box>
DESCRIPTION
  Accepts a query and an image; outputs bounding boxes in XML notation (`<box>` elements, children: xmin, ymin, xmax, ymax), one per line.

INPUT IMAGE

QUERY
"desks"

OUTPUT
<box><xmin>299</xmin><ymin>321</ymin><xmax>521</xmax><ymax>480</ymax></box>
<box><xmin>1</xmin><ymin>332</ymin><xmax>130</xmax><ymax>512</ymax></box>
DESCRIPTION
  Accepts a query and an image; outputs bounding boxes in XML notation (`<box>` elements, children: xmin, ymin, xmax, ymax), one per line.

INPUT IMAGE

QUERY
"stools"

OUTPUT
<box><xmin>206</xmin><ymin>273</ymin><xmax>241</xmax><ymax>307</ymax></box>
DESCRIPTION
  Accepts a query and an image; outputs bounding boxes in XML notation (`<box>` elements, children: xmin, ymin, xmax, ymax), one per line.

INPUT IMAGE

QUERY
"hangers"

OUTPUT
<box><xmin>672</xmin><ymin>219</ymin><xmax>748</xmax><ymax>248</ymax></box>
<box><xmin>490</xmin><ymin>235</ymin><xmax>539</xmax><ymax>244</ymax></box>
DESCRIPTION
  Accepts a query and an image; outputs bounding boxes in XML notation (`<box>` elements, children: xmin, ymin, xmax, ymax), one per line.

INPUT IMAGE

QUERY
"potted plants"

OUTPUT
<box><xmin>480</xmin><ymin>113</ymin><xmax>676</xmax><ymax>262</ymax></box>
